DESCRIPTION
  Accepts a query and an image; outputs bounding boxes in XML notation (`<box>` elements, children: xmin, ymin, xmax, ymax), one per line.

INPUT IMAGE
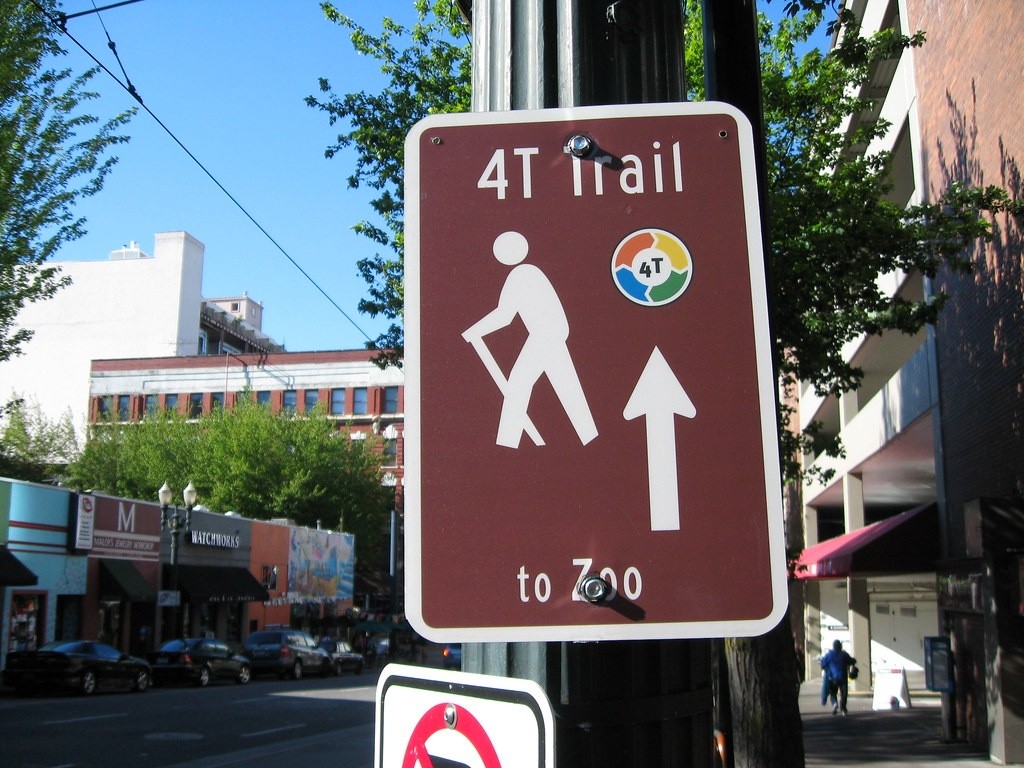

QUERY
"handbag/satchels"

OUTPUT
<box><xmin>849</xmin><ymin>662</ymin><xmax>859</xmax><ymax>679</ymax></box>
<box><xmin>367</xmin><ymin>649</ymin><xmax>372</xmax><ymax>656</ymax></box>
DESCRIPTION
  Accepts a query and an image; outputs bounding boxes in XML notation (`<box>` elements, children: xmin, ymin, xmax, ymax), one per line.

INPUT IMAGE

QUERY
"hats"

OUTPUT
<box><xmin>833</xmin><ymin>640</ymin><xmax>842</xmax><ymax>649</ymax></box>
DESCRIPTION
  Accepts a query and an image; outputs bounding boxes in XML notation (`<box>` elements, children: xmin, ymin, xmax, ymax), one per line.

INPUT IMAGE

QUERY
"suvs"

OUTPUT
<box><xmin>240</xmin><ymin>630</ymin><xmax>329</xmax><ymax>681</ymax></box>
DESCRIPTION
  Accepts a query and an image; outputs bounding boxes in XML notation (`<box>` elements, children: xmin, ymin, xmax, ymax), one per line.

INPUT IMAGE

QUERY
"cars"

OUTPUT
<box><xmin>363</xmin><ymin>633</ymin><xmax>392</xmax><ymax>668</ymax></box>
<box><xmin>319</xmin><ymin>636</ymin><xmax>366</xmax><ymax>677</ymax></box>
<box><xmin>4</xmin><ymin>638</ymin><xmax>151</xmax><ymax>699</ymax></box>
<box><xmin>148</xmin><ymin>637</ymin><xmax>252</xmax><ymax>687</ymax></box>
<box><xmin>442</xmin><ymin>643</ymin><xmax>462</xmax><ymax>668</ymax></box>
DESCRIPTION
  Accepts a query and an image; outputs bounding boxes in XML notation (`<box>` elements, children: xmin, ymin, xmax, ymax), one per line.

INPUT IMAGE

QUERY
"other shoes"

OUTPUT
<box><xmin>841</xmin><ymin>708</ymin><xmax>847</xmax><ymax>716</ymax></box>
<box><xmin>831</xmin><ymin>703</ymin><xmax>838</xmax><ymax>715</ymax></box>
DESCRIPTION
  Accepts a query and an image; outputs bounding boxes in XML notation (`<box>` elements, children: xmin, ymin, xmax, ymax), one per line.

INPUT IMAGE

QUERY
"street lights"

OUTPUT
<box><xmin>158</xmin><ymin>477</ymin><xmax>199</xmax><ymax>641</ymax></box>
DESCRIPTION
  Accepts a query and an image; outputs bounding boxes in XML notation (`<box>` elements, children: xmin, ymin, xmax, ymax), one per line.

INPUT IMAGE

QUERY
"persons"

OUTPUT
<box><xmin>819</xmin><ymin>639</ymin><xmax>856</xmax><ymax>717</ymax></box>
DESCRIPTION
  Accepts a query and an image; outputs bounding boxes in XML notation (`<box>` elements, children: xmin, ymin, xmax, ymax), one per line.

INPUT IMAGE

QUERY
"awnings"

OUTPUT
<box><xmin>787</xmin><ymin>499</ymin><xmax>938</xmax><ymax>581</ymax></box>
